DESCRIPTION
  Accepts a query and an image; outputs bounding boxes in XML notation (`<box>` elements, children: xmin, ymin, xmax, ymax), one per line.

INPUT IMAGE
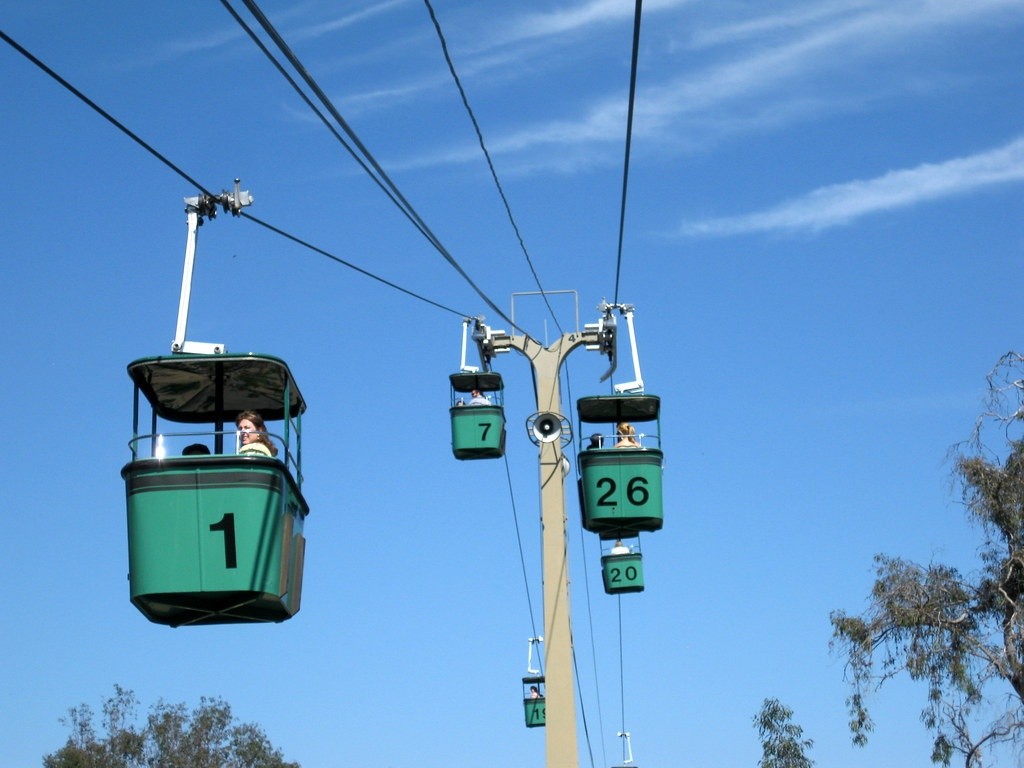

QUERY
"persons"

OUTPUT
<box><xmin>585</xmin><ymin>433</ymin><xmax>604</xmax><ymax>450</ymax></box>
<box><xmin>611</xmin><ymin>541</ymin><xmax>629</xmax><ymax>554</ymax></box>
<box><xmin>614</xmin><ymin>422</ymin><xmax>641</xmax><ymax>448</ymax></box>
<box><xmin>530</xmin><ymin>686</ymin><xmax>540</xmax><ymax>698</ymax></box>
<box><xmin>456</xmin><ymin>389</ymin><xmax>491</xmax><ymax>406</ymax></box>
<box><xmin>183</xmin><ymin>444</ymin><xmax>211</xmax><ymax>455</ymax></box>
<box><xmin>235</xmin><ymin>410</ymin><xmax>279</xmax><ymax>458</ymax></box>
